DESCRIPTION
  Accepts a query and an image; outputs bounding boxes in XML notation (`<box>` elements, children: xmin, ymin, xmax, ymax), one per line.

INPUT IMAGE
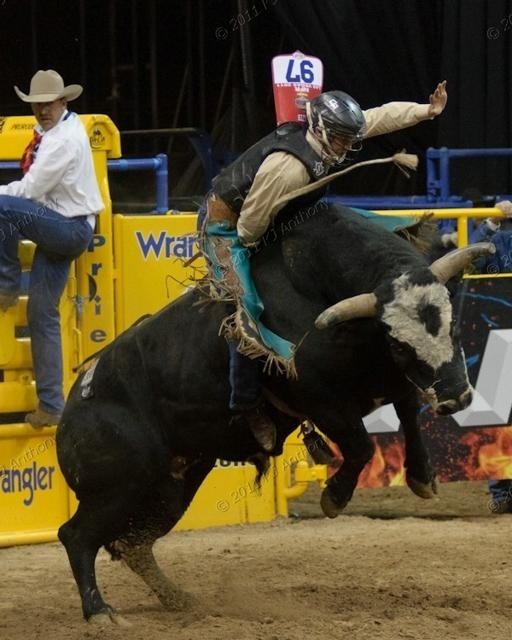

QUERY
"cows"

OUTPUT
<box><xmin>55</xmin><ymin>201</ymin><xmax>497</xmax><ymax>627</ymax></box>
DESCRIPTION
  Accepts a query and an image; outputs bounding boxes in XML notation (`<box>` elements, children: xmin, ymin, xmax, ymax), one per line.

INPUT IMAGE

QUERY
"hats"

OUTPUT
<box><xmin>14</xmin><ymin>70</ymin><xmax>83</xmax><ymax>103</ymax></box>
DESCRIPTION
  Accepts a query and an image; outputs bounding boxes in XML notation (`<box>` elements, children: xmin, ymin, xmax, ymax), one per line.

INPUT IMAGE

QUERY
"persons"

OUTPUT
<box><xmin>197</xmin><ymin>79</ymin><xmax>448</xmax><ymax>451</ymax></box>
<box><xmin>0</xmin><ymin>69</ymin><xmax>106</xmax><ymax>429</ymax></box>
<box><xmin>469</xmin><ymin>199</ymin><xmax>512</xmax><ymax>274</ymax></box>
<box><xmin>429</xmin><ymin>189</ymin><xmax>497</xmax><ymax>257</ymax></box>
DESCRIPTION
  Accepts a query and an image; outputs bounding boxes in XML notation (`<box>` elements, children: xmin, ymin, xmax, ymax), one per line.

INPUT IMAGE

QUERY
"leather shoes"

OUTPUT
<box><xmin>25</xmin><ymin>408</ymin><xmax>68</xmax><ymax>428</ymax></box>
<box><xmin>0</xmin><ymin>289</ymin><xmax>20</xmax><ymax>305</ymax></box>
<box><xmin>244</xmin><ymin>407</ymin><xmax>278</xmax><ymax>451</ymax></box>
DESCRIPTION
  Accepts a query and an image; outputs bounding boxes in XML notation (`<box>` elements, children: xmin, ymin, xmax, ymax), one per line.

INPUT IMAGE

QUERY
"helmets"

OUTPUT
<box><xmin>308</xmin><ymin>91</ymin><xmax>364</xmax><ymax>153</ymax></box>
